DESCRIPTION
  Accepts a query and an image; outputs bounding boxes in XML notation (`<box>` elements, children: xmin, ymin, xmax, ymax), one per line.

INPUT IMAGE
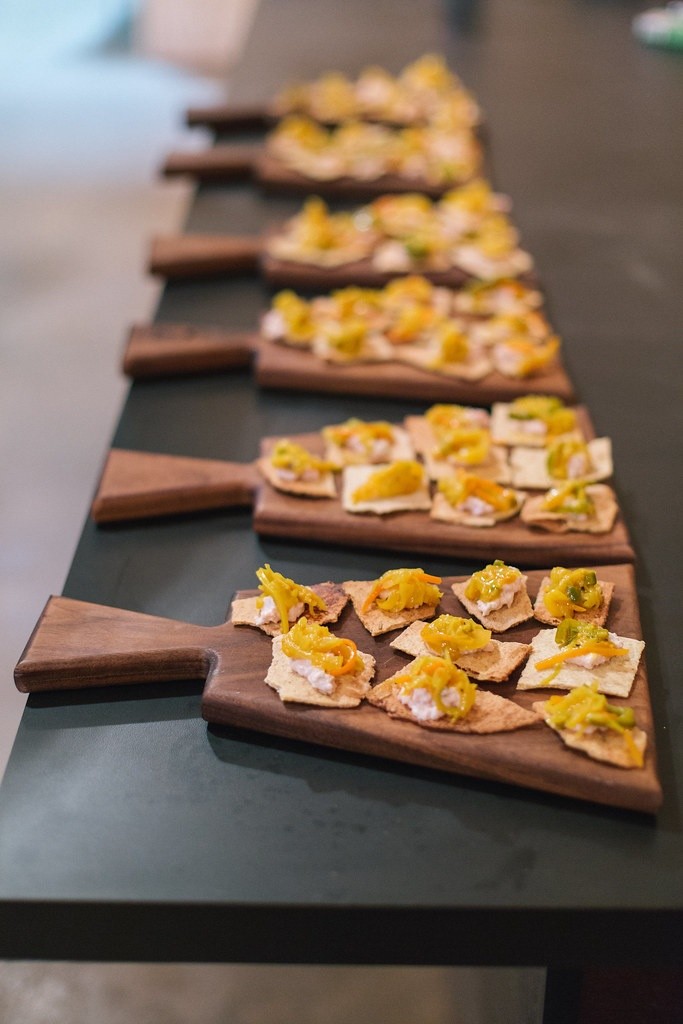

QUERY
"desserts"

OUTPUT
<box><xmin>230</xmin><ymin>70</ymin><xmax>645</xmax><ymax>770</ymax></box>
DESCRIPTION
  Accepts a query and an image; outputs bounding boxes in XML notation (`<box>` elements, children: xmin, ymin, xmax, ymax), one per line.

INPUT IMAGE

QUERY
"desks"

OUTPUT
<box><xmin>0</xmin><ymin>0</ymin><xmax>683</xmax><ymax>1024</ymax></box>
